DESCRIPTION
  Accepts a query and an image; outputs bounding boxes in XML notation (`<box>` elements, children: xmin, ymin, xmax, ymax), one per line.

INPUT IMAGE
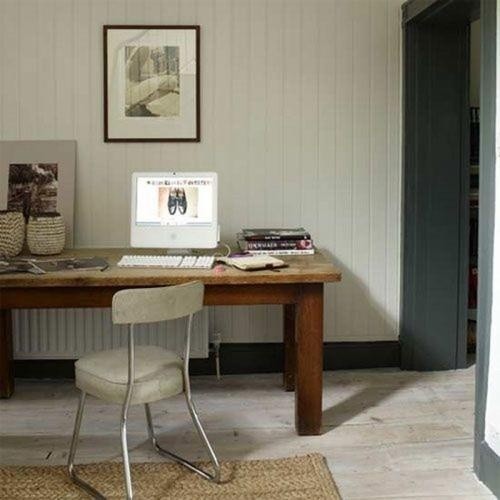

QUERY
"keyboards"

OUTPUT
<box><xmin>117</xmin><ymin>253</ymin><xmax>216</xmax><ymax>270</ymax></box>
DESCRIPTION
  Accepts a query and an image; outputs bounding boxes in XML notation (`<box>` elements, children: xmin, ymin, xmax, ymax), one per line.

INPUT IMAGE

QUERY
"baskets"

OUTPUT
<box><xmin>0</xmin><ymin>210</ymin><xmax>66</xmax><ymax>257</ymax></box>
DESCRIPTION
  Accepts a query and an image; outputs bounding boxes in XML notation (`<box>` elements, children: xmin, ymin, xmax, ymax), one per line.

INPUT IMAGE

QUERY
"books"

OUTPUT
<box><xmin>242</xmin><ymin>248</ymin><xmax>314</xmax><ymax>256</ymax></box>
<box><xmin>236</xmin><ymin>235</ymin><xmax>313</xmax><ymax>251</ymax></box>
<box><xmin>242</xmin><ymin>226</ymin><xmax>311</xmax><ymax>240</ymax></box>
<box><xmin>215</xmin><ymin>252</ymin><xmax>289</xmax><ymax>272</ymax></box>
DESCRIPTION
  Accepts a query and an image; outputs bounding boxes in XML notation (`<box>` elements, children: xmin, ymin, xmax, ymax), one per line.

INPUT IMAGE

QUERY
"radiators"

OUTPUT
<box><xmin>12</xmin><ymin>305</ymin><xmax>222</xmax><ymax>361</ymax></box>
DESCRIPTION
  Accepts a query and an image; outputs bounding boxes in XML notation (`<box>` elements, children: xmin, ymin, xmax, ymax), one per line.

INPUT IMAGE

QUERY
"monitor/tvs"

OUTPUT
<box><xmin>129</xmin><ymin>171</ymin><xmax>218</xmax><ymax>255</ymax></box>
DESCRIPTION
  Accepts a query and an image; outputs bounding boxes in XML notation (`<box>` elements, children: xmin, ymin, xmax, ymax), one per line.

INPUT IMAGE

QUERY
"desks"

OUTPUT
<box><xmin>0</xmin><ymin>248</ymin><xmax>342</xmax><ymax>436</ymax></box>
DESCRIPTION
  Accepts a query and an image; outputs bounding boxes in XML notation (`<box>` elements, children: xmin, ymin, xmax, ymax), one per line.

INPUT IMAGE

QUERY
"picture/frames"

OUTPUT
<box><xmin>104</xmin><ymin>25</ymin><xmax>200</xmax><ymax>142</ymax></box>
<box><xmin>0</xmin><ymin>139</ymin><xmax>76</xmax><ymax>250</ymax></box>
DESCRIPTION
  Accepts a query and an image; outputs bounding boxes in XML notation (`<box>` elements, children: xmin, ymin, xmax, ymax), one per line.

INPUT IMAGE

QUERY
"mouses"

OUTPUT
<box><xmin>214</xmin><ymin>264</ymin><xmax>224</xmax><ymax>272</ymax></box>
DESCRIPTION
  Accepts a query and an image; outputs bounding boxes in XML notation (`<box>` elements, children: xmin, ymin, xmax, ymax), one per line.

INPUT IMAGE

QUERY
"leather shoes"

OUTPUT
<box><xmin>167</xmin><ymin>187</ymin><xmax>187</xmax><ymax>215</ymax></box>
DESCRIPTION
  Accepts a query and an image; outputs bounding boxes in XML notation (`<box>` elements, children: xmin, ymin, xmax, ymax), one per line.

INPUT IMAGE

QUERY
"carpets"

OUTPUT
<box><xmin>0</xmin><ymin>452</ymin><xmax>342</xmax><ymax>500</ymax></box>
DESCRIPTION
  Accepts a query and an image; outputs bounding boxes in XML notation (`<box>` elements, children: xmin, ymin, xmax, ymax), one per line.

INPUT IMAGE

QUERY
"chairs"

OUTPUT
<box><xmin>67</xmin><ymin>280</ymin><xmax>221</xmax><ymax>500</ymax></box>
